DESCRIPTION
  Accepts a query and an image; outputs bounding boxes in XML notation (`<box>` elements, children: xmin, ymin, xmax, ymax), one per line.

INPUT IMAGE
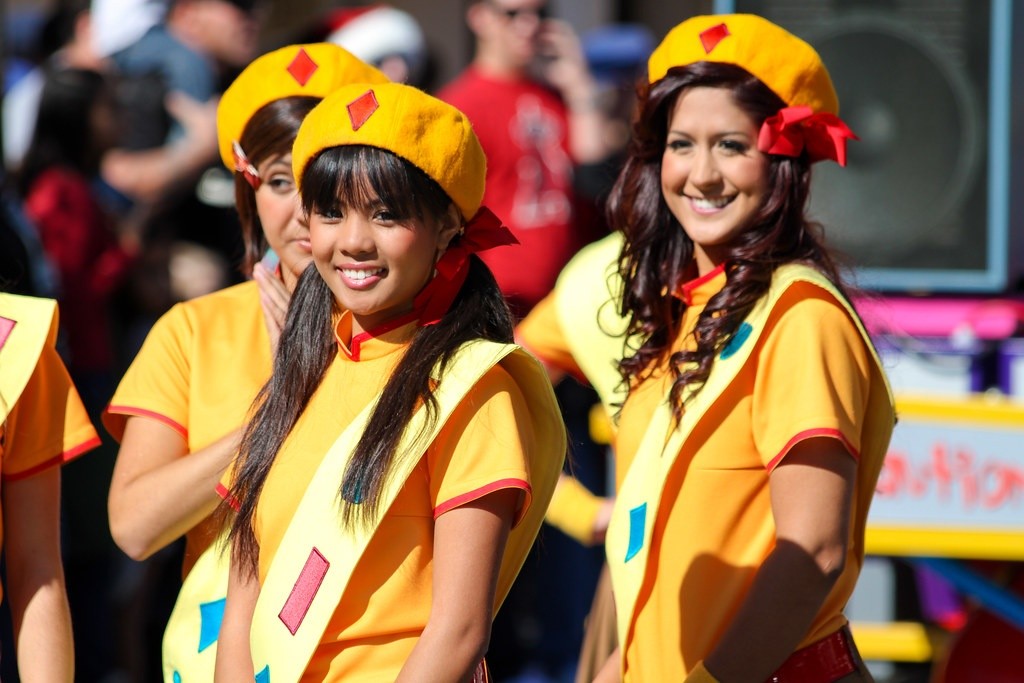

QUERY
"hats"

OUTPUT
<box><xmin>320</xmin><ymin>4</ymin><xmax>425</xmax><ymax>75</ymax></box>
<box><xmin>215</xmin><ymin>43</ymin><xmax>392</xmax><ymax>174</ymax></box>
<box><xmin>645</xmin><ymin>15</ymin><xmax>855</xmax><ymax>169</ymax></box>
<box><xmin>292</xmin><ymin>80</ymin><xmax>487</xmax><ymax>223</ymax></box>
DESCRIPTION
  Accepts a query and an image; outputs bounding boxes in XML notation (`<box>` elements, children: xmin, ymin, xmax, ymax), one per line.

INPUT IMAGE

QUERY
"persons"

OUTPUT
<box><xmin>595</xmin><ymin>14</ymin><xmax>898</xmax><ymax>683</ymax></box>
<box><xmin>214</xmin><ymin>80</ymin><xmax>569</xmax><ymax>683</ymax></box>
<box><xmin>513</xmin><ymin>230</ymin><xmax>653</xmax><ymax>683</ymax></box>
<box><xmin>102</xmin><ymin>43</ymin><xmax>387</xmax><ymax>579</ymax></box>
<box><xmin>0</xmin><ymin>291</ymin><xmax>103</xmax><ymax>683</ymax></box>
<box><xmin>0</xmin><ymin>0</ymin><xmax>612</xmax><ymax>368</ymax></box>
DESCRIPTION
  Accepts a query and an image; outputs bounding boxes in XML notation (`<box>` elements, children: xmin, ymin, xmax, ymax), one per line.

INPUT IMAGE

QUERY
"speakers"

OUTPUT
<box><xmin>709</xmin><ymin>0</ymin><xmax>1024</xmax><ymax>294</ymax></box>
